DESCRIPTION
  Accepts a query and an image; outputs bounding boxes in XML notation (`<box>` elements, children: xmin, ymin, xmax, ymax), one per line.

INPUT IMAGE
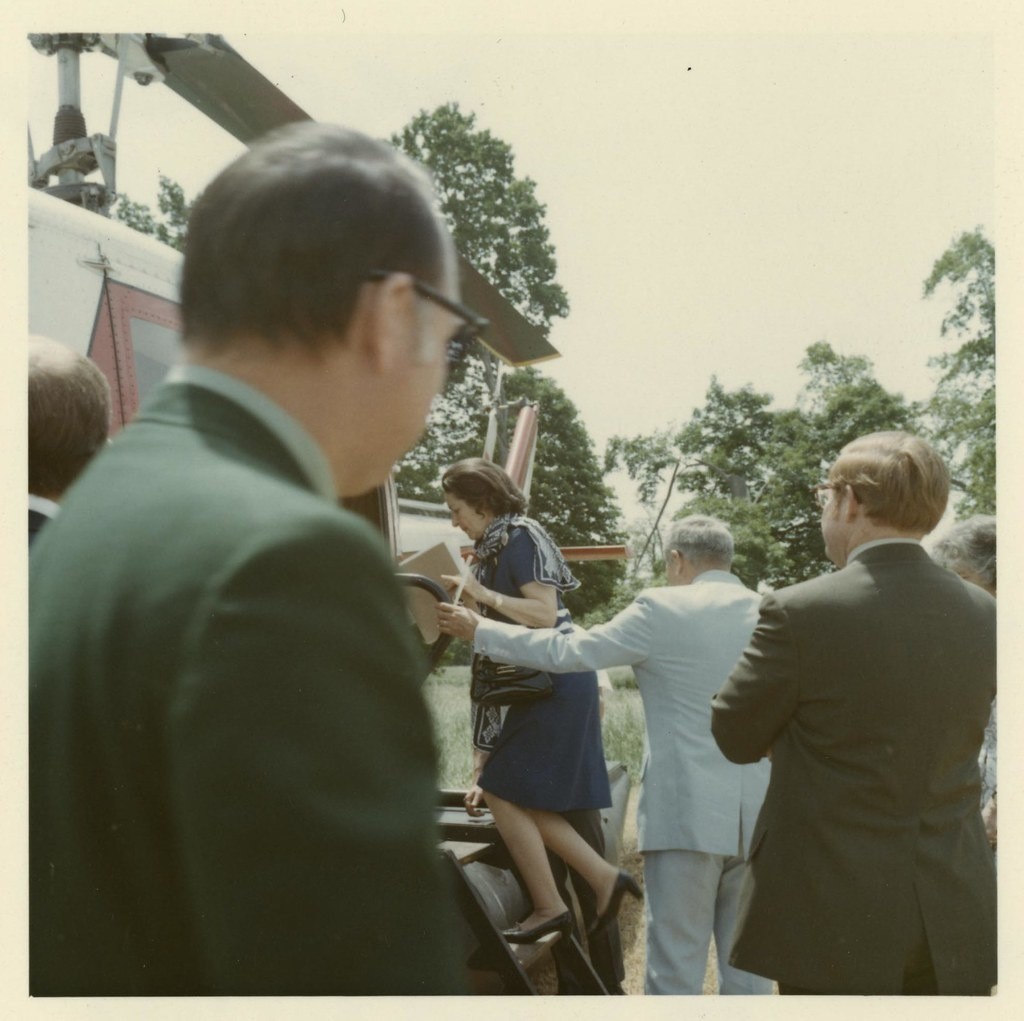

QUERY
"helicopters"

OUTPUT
<box><xmin>25</xmin><ymin>33</ymin><xmax>633</xmax><ymax>976</ymax></box>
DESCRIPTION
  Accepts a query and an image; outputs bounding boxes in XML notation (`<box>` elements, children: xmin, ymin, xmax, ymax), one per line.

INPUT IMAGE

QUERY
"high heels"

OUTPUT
<box><xmin>587</xmin><ymin>870</ymin><xmax>643</xmax><ymax>937</ymax></box>
<box><xmin>502</xmin><ymin>911</ymin><xmax>571</xmax><ymax>946</ymax></box>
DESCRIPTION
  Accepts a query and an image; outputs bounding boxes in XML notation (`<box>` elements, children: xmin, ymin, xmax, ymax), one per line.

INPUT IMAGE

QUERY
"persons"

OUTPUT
<box><xmin>30</xmin><ymin>114</ymin><xmax>468</xmax><ymax>1001</ymax></box>
<box><xmin>707</xmin><ymin>429</ymin><xmax>995</xmax><ymax>998</ymax></box>
<box><xmin>29</xmin><ymin>334</ymin><xmax>112</xmax><ymax>554</ymax></box>
<box><xmin>440</xmin><ymin>456</ymin><xmax>642</xmax><ymax>942</ymax></box>
<box><xmin>928</xmin><ymin>510</ymin><xmax>995</xmax><ymax>851</ymax></box>
<box><xmin>434</xmin><ymin>513</ymin><xmax>776</xmax><ymax>997</ymax></box>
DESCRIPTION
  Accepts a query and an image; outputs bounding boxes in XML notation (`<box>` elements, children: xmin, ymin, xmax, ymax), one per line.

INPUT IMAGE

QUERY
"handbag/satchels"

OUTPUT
<box><xmin>470</xmin><ymin>649</ymin><xmax>552</xmax><ymax>703</ymax></box>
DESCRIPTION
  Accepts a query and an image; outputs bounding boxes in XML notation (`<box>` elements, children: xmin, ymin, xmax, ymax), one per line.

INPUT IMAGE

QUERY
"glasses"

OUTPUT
<box><xmin>818</xmin><ymin>487</ymin><xmax>830</xmax><ymax>508</ymax></box>
<box><xmin>360</xmin><ymin>268</ymin><xmax>486</xmax><ymax>363</ymax></box>
